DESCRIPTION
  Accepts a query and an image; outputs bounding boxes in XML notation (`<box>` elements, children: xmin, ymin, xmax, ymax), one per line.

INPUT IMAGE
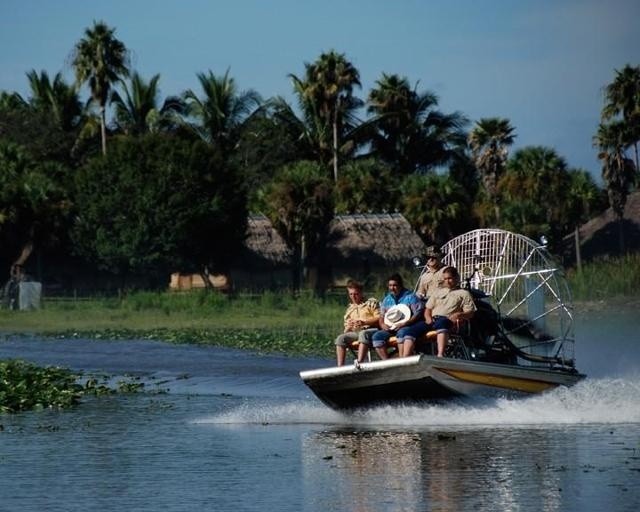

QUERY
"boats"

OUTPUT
<box><xmin>302</xmin><ymin>330</ymin><xmax>585</xmax><ymax>415</ymax></box>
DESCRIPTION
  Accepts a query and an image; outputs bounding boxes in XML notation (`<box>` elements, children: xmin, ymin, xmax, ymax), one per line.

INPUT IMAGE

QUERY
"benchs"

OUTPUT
<box><xmin>351</xmin><ymin>299</ymin><xmax>472</xmax><ymax>362</ymax></box>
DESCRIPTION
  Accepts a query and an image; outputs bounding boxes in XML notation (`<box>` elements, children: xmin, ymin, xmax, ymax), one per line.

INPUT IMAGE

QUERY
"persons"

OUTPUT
<box><xmin>415</xmin><ymin>245</ymin><xmax>448</xmax><ymax>300</ymax></box>
<box><xmin>333</xmin><ymin>279</ymin><xmax>380</xmax><ymax>367</ymax></box>
<box><xmin>401</xmin><ymin>267</ymin><xmax>478</xmax><ymax>358</ymax></box>
<box><xmin>372</xmin><ymin>272</ymin><xmax>422</xmax><ymax>360</ymax></box>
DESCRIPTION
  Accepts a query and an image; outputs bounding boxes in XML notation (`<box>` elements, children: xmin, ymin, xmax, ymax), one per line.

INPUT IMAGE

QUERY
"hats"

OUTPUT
<box><xmin>384</xmin><ymin>304</ymin><xmax>411</xmax><ymax>327</ymax></box>
<box><xmin>421</xmin><ymin>245</ymin><xmax>445</xmax><ymax>258</ymax></box>
